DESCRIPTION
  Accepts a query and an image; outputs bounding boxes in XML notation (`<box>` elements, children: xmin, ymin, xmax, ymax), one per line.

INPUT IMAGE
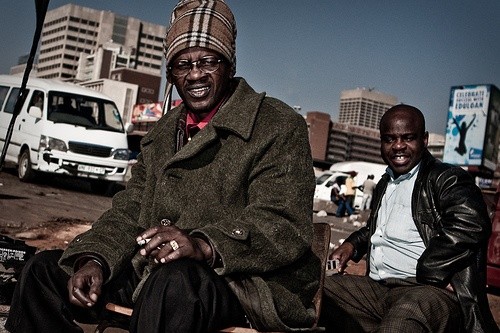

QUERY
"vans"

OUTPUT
<box><xmin>0</xmin><ymin>74</ymin><xmax>135</xmax><ymax>196</ymax></box>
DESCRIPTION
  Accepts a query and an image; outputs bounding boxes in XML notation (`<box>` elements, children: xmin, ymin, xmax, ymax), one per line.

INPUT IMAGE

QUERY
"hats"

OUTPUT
<box><xmin>164</xmin><ymin>0</ymin><xmax>237</xmax><ymax>76</ymax></box>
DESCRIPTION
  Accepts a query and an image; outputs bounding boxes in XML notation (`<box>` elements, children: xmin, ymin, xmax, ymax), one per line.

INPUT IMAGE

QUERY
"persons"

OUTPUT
<box><xmin>345</xmin><ymin>171</ymin><xmax>360</xmax><ymax>216</ymax></box>
<box><xmin>331</xmin><ymin>177</ymin><xmax>353</xmax><ymax>218</ymax></box>
<box><xmin>5</xmin><ymin>0</ymin><xmax>322</xmax><ymax>333</ymax></box>
<box><xmin>323</xmin><ymin>104</ymin><xmax>500</xmax><ymax>333</ymax></box>
<box><xmin>360</xmin><ymin>175</ymin><xmax>376</xmax><ymax>212</ymax></box>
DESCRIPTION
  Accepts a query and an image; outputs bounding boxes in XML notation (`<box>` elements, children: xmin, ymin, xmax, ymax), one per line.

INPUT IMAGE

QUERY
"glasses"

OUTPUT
<box><xmin>167</xmin><ymin>56</ymin><xmax>224</xmax><ymax>75</ymax></box>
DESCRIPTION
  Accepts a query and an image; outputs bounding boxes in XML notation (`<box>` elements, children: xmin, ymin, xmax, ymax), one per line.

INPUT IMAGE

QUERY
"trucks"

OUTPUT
<box><xmin>314</xmin><ymin>161</ymin><xmax>388</xmax><ymax>209</ymax></box>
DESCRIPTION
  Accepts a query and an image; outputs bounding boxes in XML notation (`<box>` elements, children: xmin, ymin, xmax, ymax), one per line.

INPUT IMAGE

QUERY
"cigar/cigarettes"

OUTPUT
<box><xmin>139</xmin><ymin>239</ymin><xmax>151</xmax><ymax>246</ymax></box>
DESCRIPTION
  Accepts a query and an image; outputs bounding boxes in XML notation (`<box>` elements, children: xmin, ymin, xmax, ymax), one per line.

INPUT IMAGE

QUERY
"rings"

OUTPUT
<box><xmin>170</xmin><ymin>240</ymin><xmax>178</xmax><ymax>251</ymax></box>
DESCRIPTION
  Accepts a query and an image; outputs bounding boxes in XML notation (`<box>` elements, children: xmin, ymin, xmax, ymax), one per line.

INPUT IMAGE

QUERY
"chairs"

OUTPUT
<box><xmin>105</xmin><ymin>223</ymin><xmax>331</xmax><ymax>333</ymax></box>
